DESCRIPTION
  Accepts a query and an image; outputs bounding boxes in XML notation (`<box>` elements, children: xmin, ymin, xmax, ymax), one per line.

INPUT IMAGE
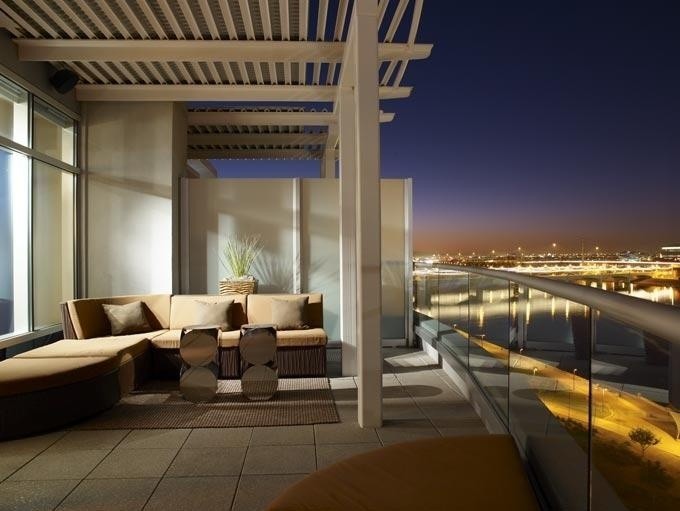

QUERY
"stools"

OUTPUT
<box><xmin>0</xmin><ymin>357</ymin><xmax>120</xmax><ymax>441</ymax></box>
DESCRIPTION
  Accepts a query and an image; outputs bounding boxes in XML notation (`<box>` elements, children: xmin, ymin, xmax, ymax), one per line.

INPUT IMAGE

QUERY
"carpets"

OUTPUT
<box><xmin>80</xmin><ymin>375</ymin><xmax>341</xmax><ymax>429</ymax></box>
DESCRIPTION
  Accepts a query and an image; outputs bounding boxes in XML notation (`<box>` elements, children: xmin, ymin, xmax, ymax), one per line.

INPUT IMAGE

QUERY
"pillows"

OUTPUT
<box><xmin>101</xmin><ymin>301</ymin><xmax>152</xmax><ymax>334</ymax></box>
<box><xmin>169</xmin><ymin>297</ymin><xmax>234</xmax><ymax>330</ymax></box>
<box><xmin>267</xmin><ymin>297</ymin><xmax>311</xmax><ymax>331</ymax></box>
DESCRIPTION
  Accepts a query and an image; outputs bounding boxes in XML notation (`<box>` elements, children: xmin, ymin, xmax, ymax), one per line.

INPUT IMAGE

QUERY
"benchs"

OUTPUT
<box><xmin>268</xmin><ymin>432</ymin><xmax>541</xmax><ymax>511</ymax></box>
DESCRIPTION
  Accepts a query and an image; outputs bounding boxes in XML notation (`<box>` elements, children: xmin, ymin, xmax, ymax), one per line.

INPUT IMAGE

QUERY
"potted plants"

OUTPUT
<box><xmin>217</xmin><ymin>232</ymin><xmax>268</xmax><ymax>295</ymax></box>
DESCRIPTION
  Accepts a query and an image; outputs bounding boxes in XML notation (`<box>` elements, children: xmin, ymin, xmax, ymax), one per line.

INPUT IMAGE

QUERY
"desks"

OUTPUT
<box><xmin>239</xmin><ymin>324</ymin><xmax>278</xmax><ymax>400</ymax></box>
<box><xmin>179</xmin><ymin>325</ymin><xmax>222</xmax><ymax>401</ymax></box>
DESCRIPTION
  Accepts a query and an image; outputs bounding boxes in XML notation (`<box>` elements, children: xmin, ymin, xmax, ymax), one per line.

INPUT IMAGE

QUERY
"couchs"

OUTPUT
<box><xmin>11</xmin><ymin>294</ymin><xmax>328</xmax><ymax>395</ymax></box>
